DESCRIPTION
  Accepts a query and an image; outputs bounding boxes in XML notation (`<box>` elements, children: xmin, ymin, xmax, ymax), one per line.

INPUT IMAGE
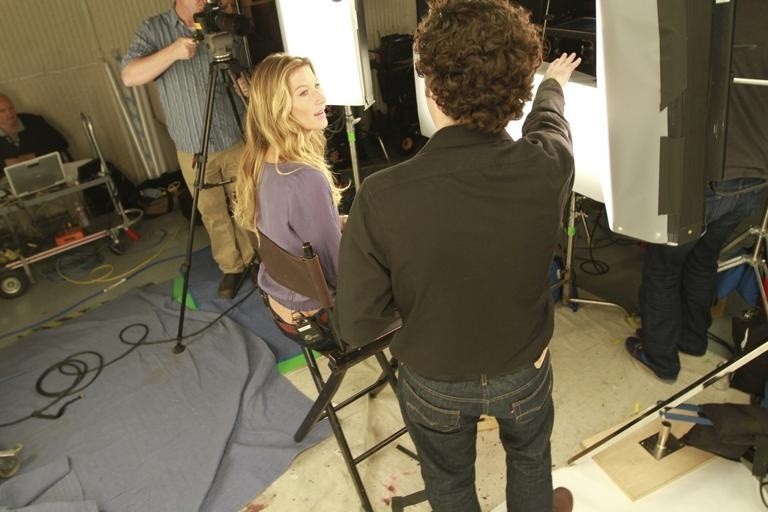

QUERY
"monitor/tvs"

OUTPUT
<box><xmin>3</xmin><ymin>151</ymin><xmax>67</xmax><ymax>200</ymax></box>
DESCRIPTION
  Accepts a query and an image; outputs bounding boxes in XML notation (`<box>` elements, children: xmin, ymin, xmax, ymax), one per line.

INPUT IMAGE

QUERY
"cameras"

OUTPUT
<box><xmin>193</xmin><ymin>2</ymin><xmax>254</xmax><ymax>37</ymax></box>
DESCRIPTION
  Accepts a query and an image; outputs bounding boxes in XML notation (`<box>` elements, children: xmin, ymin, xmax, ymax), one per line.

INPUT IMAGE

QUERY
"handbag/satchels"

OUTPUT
<box><xmin>136</xmin><ymin>171</ymin><xmax>188</xmax><ymax>216</ymax></box>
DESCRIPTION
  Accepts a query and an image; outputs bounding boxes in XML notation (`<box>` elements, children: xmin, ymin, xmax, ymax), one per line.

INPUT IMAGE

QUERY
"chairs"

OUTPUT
<box><xmin>245</xmin><ymin>222</ymin><xmax>409</xmax><ymax>512</ymax></box>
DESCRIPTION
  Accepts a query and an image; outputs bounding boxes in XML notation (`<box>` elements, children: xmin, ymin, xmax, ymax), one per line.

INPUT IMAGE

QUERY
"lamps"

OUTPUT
<box><xmin>274</xmin><ymin>0</ymin><xmax>377</xmax><ymax>193</ymax></box>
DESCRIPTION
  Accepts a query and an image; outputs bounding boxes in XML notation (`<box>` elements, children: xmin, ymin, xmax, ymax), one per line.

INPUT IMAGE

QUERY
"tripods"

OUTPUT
<box><xmin>173</xmin><ymin>57</ymin><xmax>253</xmax><ymax>353</ymax></box>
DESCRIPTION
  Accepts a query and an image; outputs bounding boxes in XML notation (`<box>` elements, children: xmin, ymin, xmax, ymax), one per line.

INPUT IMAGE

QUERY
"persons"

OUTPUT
<box><xmin>333</xmin><ymin>1</ymin><xmax>581</xmax><ymax>512</ymax></box>
<box><xmin>624</xmin><ymin>2</ymin><xmax>768</xmax><ymax>384</ymax></box>
<box><xmin>0</xmin><ymin>94</ymin><xmax>69</xmax><ymax>177</ymax></box>
<box><xmin>232</xmin><ymin>52</ymin><xmax>343</xmax><ymax>351</ymax></box>
<box><xmin>120</xmin><ymin>1</ymin><xmax>270</xmax><ymax>299</ymax></box>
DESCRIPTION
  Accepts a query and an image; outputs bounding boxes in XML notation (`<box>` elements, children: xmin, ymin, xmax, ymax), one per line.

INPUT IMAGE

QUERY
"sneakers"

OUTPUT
<box><xmin>218</xmin><ymin>273</ymin><xmax>240</xmax><ymax>298</ymax></box>
<box><xmin>247</xmin><ymin>264</ymin><xmax>259</xmax><ymax>284</ymax></box>
<box><xmin>553</xmin><ymin>487</ymin><xmax>573</xmax><ymax>511</ymax></box>
<box><xmin>626</xmin><ymin>328</ymin><xmax>663</xmax><ymax>378</ymax></box>
